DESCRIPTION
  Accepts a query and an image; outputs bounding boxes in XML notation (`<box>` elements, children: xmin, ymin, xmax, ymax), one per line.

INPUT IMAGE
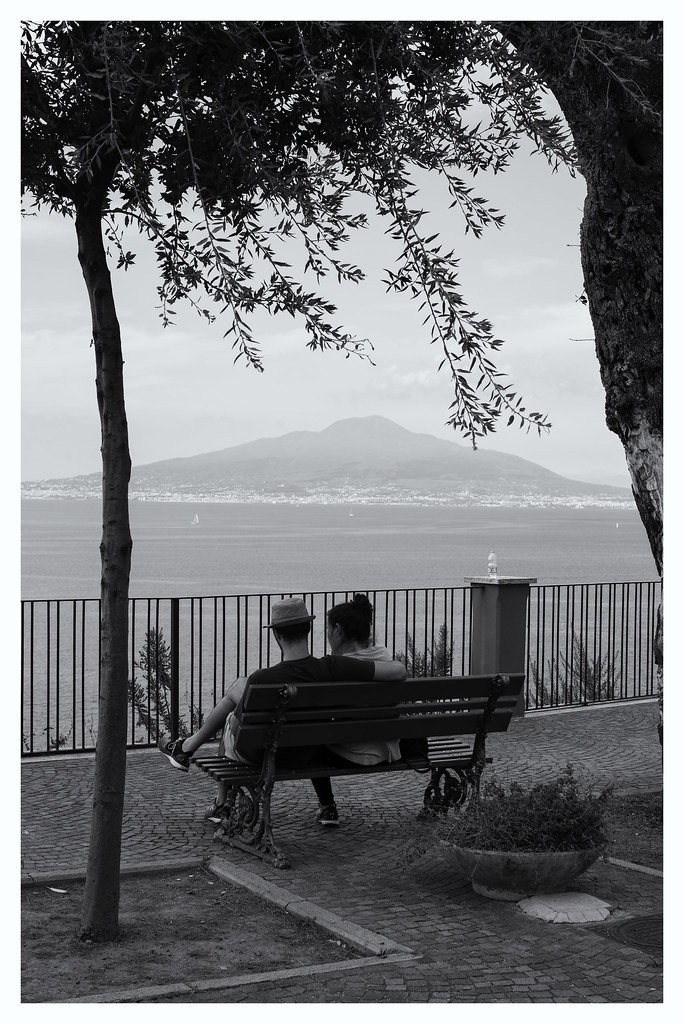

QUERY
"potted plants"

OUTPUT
<box><xmin>391</xmin><ymin>773</ymin><xmax>623</xmax><ymax>900</ymax></box>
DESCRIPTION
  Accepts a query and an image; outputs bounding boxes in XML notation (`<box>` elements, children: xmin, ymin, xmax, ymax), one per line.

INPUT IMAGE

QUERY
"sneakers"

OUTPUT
<box><xmin>160</xmin><ymin>737</ymin><xmax>189</xmax><ymax>773</ymax></box>
<box><xmin>206</xmin><ymin>796</ymin><xmax>223</xmax><ymax>823</ymax></box>
<box><xmin>315</xmin><ymin>802</ymin><xmax>340</xmax><ymax>826</ymax></box>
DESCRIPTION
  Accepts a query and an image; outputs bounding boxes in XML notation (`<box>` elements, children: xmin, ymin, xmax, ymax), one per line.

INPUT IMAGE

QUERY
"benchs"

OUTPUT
<box><xmin>187</xmin><ymin>673</ymin><xmax>527</xmax><ymax>866</ymax></box>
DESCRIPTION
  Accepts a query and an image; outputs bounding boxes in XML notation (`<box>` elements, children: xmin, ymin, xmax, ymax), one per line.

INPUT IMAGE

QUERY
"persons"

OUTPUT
<box><xmin>310</xmin><ymin>593</ymin><xmax>401</xmax><ymax>827</ymax></box>
<box><xmin>159</xmin><ymin>598</ymin><xmax>407</xmax><ymax>824</ymax></box>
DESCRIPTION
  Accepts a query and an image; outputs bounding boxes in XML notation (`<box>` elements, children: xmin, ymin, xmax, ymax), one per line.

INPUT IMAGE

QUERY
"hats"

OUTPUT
<box><xmin>262</xmin><ymin>598</ymin><xmax>316</xmax><ymax>629</ymax></box>
